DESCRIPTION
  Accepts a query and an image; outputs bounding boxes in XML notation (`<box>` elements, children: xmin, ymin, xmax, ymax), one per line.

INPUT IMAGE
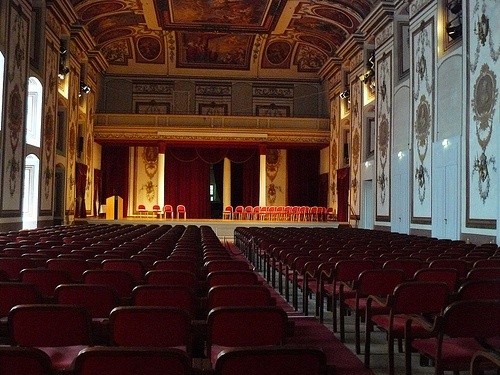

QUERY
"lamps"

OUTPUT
<box><xmin>60</xmin><ymin>43</ymin><xmax>68</xmax><ymax>54</ymax></box>
<box><xmin>359</xmin><ymin>68</ymin><xmax>375</xmax><ymax>84</ymax></box>
<box><xmin>339</xmin><ymin>89</ymin><xmax>350</xmax><ymax>100</ymax></box>
<box><xmin>57</xmin><ymin>66</ymin><xmax>70</xmax><ymax>79</ymax></box>
<box><xmin>80</xmin><ymin>81</ymin><xmax>91</xmax><ymax>94</ymax></box>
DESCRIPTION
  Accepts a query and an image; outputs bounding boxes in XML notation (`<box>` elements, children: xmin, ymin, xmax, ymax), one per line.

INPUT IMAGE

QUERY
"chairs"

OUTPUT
<box><xmin>176</xmin><ymin>205</ymin><xmax>186</xmax><ymax>219</ymax></box>
<box><xmin>152</xmin><ymin>205</ymin><xmax>162</xmax><ymax>219</ymax></box>
<box><xmin>0</xmin><ymin>223</ymin><xmax>500</xmax><ymax>375</ymax></box>
<box><xmin>164</xmin><ymin>205</ymin><xmax>173</xmax><ymax>219</ymax></box>
<box><xmin>137</xmin><ymin>205</ymin><xmax>148</xmax><ymax>219</ymax></box>
<box><xmin>222</xmin><ymin>204</ymin><xmax>337</xmax><ymax>222</ymax></box>
